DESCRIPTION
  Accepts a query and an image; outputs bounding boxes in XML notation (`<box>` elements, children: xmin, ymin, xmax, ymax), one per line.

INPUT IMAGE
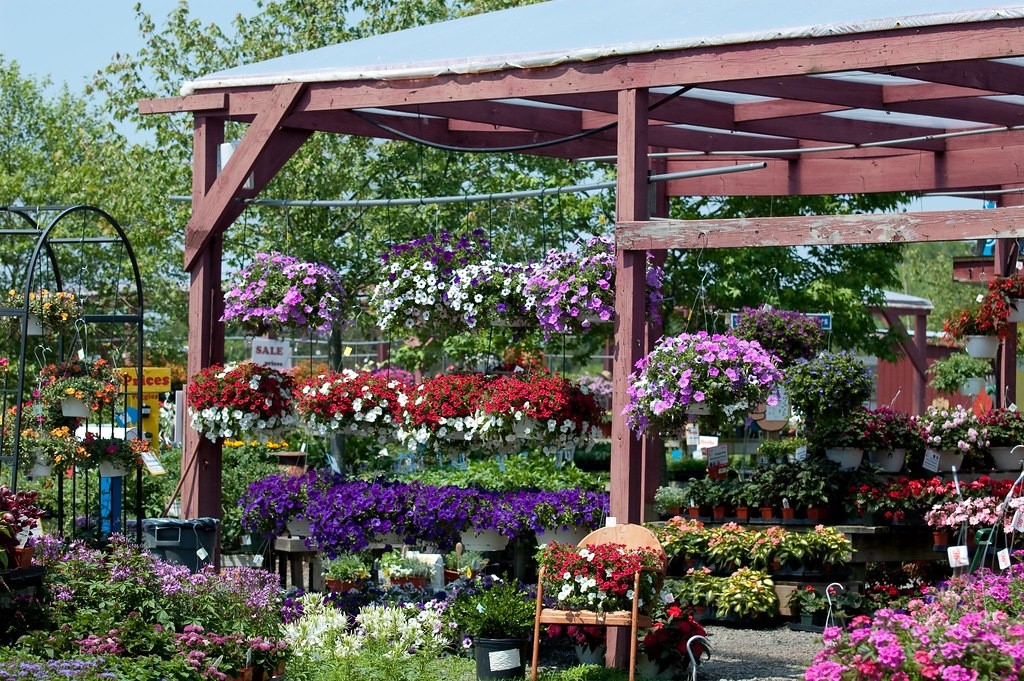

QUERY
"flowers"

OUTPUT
<box><xmin>1</xmin><ymin>220</ymin><xmax>1022</xmax><ymax>679</ymax></box>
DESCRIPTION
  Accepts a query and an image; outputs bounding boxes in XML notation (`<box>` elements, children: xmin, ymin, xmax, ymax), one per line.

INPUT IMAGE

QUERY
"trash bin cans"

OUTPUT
<box><xmin>140</xmin><ymin>517</ymin><xmax>222</xmax><ymax>575</ymax></box>
